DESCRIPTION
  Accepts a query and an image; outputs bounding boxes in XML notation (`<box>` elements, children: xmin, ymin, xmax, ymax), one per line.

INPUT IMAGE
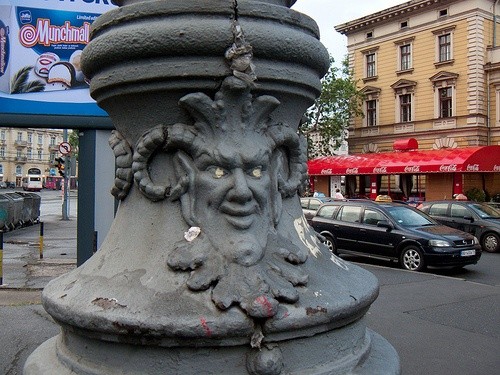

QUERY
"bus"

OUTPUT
<box><xmin>46</xmin><ymin>176</ymin><xmax>75</xmax><ymax>190</ymax></box>
<box><xmin>21</xmin><ymin>175</ymin><xmax>42</xmax><ymax>192</ymax></box>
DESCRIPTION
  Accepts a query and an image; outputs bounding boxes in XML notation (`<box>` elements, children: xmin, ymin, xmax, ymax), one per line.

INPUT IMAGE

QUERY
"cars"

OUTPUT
<box><xmin>311</xmin><ymin>199</ymin><xmax>482</xmax><ymax>271</ymax></box>
<box><xmin>300</xmin><ymin>197</ymin><xmax>335</xmax><ymax>225</ymax></box>
<box><xmin>417</xmin><ymin>200</ymin><xmax>500</xmax><ymax>252</ymax></box>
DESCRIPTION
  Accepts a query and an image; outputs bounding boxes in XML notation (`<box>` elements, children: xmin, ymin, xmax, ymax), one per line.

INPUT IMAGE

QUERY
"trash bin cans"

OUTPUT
<box><xmin>0</xmin><ymin>192</ymin><xmax>41</xmax><ymax>229</ymax></box>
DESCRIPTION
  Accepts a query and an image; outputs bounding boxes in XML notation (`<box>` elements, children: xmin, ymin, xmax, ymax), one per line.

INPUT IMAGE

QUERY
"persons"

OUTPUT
<box><xmin>334</xmin><ymin>189</ymin><xmax>344</xmax><ymax>200</ymax></box>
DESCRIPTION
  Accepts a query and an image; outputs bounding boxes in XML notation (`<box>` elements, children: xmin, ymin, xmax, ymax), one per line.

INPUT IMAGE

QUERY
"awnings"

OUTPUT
<box><xmin>307</xmin><ymin>145</ymin><xmax>500</xmax><ymax>175</ymax></box>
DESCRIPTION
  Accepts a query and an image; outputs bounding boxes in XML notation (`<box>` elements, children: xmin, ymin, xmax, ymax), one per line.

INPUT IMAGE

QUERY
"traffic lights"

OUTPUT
<box><xmin>55</xmin><ymin>157</ymin><xmax>59</xmax><ymax>168</ymax></box>
<box><xmin>59</xmin><ymin>156</ymin><xmax>66</xmax><ymax>176</ymax></box>
